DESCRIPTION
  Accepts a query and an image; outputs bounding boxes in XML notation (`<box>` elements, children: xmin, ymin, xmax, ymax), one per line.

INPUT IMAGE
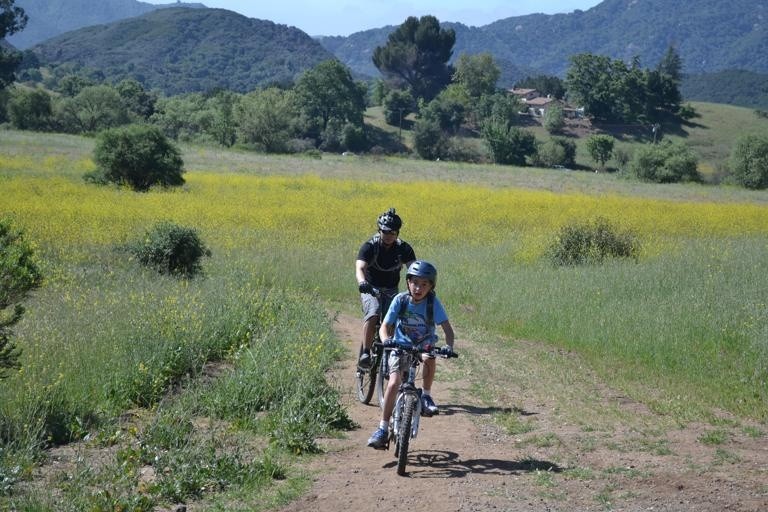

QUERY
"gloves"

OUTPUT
<box><xmin>359</xmin><ymin>280</ymin><xmax>373</xmax><ymax>295</ymax></box>
<box><xmin>440</xmin><ymin>344</ymin><xmax>453</xmax><ymax>359</ymax></box>
<box><xmin>382</xmin><ymin>338</ymin><xmax>399</xmax><ymax>348</ymax></box>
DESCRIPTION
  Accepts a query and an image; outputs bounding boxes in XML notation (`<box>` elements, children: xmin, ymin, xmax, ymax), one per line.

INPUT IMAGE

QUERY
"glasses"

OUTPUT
<box><xmin>380</xmin><ymin>229</ymin><xmax>397</xmax><ymax>235</ymax></box>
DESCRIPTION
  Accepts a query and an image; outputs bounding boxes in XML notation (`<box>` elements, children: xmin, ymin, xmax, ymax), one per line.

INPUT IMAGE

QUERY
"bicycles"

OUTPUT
<box><xmin>370</xmin><ymin>340</ymin><xmax>459</xmax><ymax>480</ymax></box>
<box><xmin>355</xmin><ymin>287</ymin><xmax>400</xmax><ymax>404</ymax></box>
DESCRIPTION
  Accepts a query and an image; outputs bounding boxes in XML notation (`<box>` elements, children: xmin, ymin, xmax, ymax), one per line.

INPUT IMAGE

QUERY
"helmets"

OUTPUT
<box><xmin>377</xmin><ymin>211</ymin><xmax>402</xmax><ymax>231</ymax></box>
<box><xmin>406</xmin><ymin>260</ymin><xmax>437</xmax><ymax>288</ymax></box>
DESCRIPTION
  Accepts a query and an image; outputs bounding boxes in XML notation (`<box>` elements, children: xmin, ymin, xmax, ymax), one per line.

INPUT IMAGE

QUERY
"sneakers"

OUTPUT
<box><xmin>421</xmin><ymin>393</ymin><xmax>439</xmax><ymax>415</ymax></box>
<box><xmin>359</xmin><ymin>353</ymin><xmax>371</xmax><ymax>367</ymax></box>
<box><xmin>367</xmin><ymin>427</ymin><xmax>389</xmax><ymax>449</ymax></box>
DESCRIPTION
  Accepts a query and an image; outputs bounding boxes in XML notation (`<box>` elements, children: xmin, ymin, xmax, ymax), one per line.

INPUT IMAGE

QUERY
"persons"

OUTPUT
<box><xmin>354</xmin><ymin>211</ymin><xmax>417</xmax><ymax>380</ymax></box>
<box><xmin>367</xmin><ymin>260</ymin><xmax>455</xmax><ymax>447</ymax></box>
